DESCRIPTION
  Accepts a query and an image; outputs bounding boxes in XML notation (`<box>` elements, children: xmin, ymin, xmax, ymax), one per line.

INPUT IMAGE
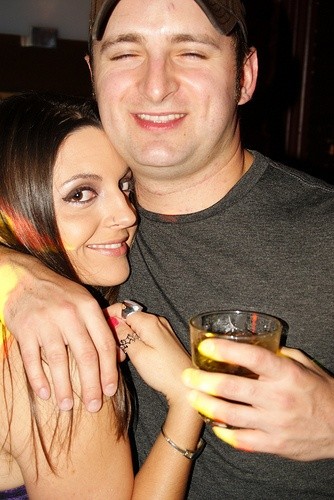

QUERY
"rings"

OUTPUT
<box><xmin>118</xmin><ymin>331</ymin><xmax>140</xmax><ymax>352</ymax></box>
<box><xmin>121</xmin><ymin>300</ymin><xmax>144</xmax><ymax>320</ymax></box>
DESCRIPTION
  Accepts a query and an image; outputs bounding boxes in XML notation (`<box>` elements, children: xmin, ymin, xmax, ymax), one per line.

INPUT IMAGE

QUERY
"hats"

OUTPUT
<box><xmin>89</xmin><ymin>0</ymin><xmax>248</xmax><ymax>43</ymax></box>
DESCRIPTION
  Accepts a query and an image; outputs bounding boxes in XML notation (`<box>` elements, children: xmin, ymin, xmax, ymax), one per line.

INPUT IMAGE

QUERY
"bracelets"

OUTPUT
<box><xmin>160</xmin><ymin>424</ymin><xmax>206</xmax><ymax>462</ymax></box>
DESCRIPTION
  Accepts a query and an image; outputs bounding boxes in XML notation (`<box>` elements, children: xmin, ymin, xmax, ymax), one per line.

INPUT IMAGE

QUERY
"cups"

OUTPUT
<box><xmin>187</xmin><ymin>310</ymin><xmax>285</xmax><ymax>430</ymax></box>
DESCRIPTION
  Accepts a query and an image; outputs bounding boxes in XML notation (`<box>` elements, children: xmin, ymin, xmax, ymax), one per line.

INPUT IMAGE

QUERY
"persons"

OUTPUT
<box><xmin>2</xmin><ymin>94</ymin><xmax>204</xmax><ymax>500</ymax></box>
<box><xmin>1</xmin><ymin>0</ymin><xmax>334</xmax><ymax>500</ymax></box>
